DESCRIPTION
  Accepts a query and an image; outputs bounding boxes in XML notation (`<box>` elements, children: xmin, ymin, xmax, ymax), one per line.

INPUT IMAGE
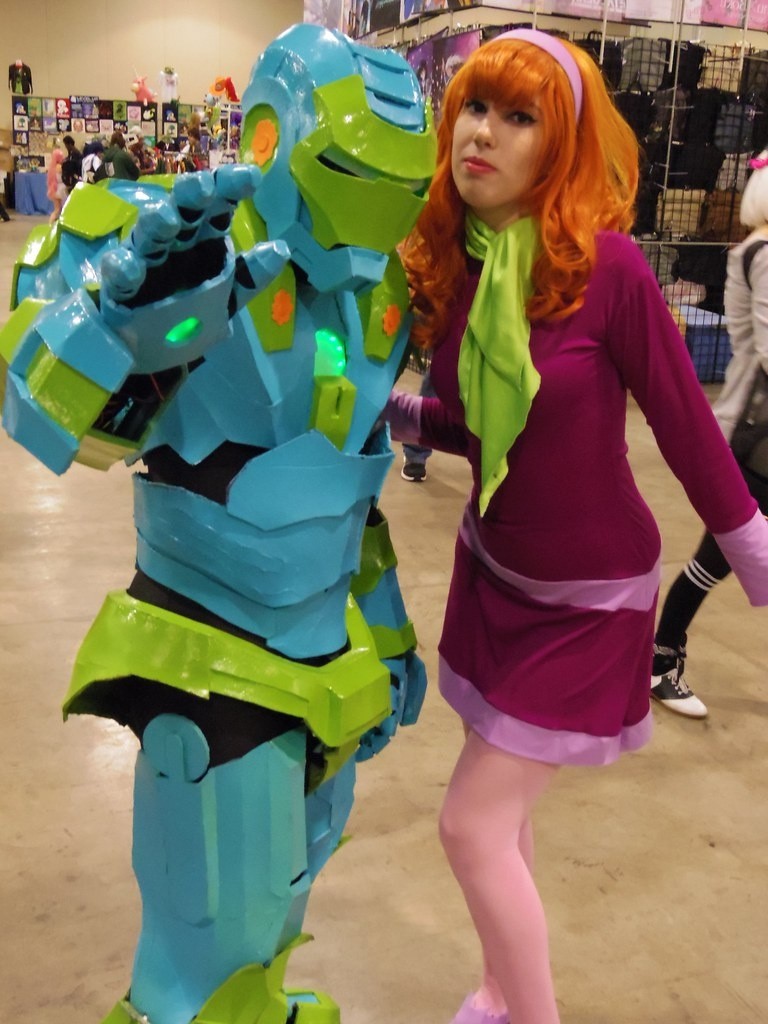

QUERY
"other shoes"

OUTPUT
<box><xmin>449</xmin><ymin>994</ymin><xmax>510</xmax><ymax>1024</ymax></box>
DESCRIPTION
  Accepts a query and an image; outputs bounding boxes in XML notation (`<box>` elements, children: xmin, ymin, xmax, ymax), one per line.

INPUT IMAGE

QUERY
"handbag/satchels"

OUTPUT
<box><xmin>615</xmin><ymin>36</ymin><xmax>668</xmax><ymax>92</ymax></box>
<box><xmin>93</xmin><ymin>160</ymin><xmax>108</xmax><ymax>183</ymax></box>
<box><xmin>570</xmin><ymin>29</ymin><xmax>622</xmax><ymax>92</ymax></box>
<box><xmin>729</xmin><ymin>364</ymin><xmax>768</xmax><ymax>488</ymax></box>
<box><xmin>684</xmin><ymin>305</ymin><xmax>734</xmax><ymax>384</ymax></box>
<box><xmin>54</xmin><ymin>182</ymin><xmax>68</xmax><ymax>200</ymax></box>
<box><xmin>613</xmin><ymin>80</ymin><xmax>768</xmax><ymax>305</ymax></box>
<box><xmin>740</xmin><ymin>50</ymin><xmax>768</xmax><ymax>107</ymax></box>
<box><xmin>659</xmin><ymin>37</ymin><xmax>713</xmax><ymax>92</ymax></box>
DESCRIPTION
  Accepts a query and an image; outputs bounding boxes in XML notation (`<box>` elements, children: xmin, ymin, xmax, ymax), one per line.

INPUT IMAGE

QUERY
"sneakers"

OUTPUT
<box><xmin>651</xmin><ymin>668</ymin><xmax>709</xmax><ymax>719</ymax></box>
<box><xmin>401</xmin><ymin>453</ymin><xmax>428</xmax><ymax>482</ymax></box>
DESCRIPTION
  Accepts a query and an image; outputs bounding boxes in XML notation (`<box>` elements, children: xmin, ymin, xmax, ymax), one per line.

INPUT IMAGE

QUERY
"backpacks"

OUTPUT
<box><xmin>86</xmin><ymin>155</ymin><xmax>97</xmax><ymax>184</ymax></box>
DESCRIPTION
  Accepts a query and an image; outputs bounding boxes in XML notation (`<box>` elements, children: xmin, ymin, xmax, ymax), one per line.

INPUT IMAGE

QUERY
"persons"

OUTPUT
<box><xmin>81</xmin><ymin>141</ymin><xmax>103</xmax><ymax>185</ymax></box>
<box><xmin>157</xmin><ymin>64</ymin><xmax>180</xmax><ymax>102</ymax></box>
<box><xmin>648</xmin><ymin>146</ymin><xmax>768</xmax><ymax>719</ymax></box>
<box><xmin>45</xmin><ymin>149</ymin><xmax>70</xmax><ymax>226</ymax></box>
<box><xmin>0</xmin><ymin>22</ymin><xmax>440</xmax><ymax>1024</ymax></box>
<box><xmin>400</xmin><ymin>352</ymin><xmax>439</xmax><ymax>483</ymax></box>
<box><xmin>0</xmin><ymin>202</ymin><xmax>10</xmax><ymax>222</ymax></box>
<box><xmin>379</xmin><ymin>29</ymin><xmax>768</xmax><ymax>1024</ymax></box>
<box><xmin>188</xmin><ymin>127</ymin><xmax>206</xmax><ymax>161</ymax></box>
<box><xmin>94</xmin><ymin>131</ymin><xmax>141</xmax><ymax>183</ymax></box>
<box><xmin>6</xmin><ymin>59</ymin><xmax>33</xmax><ymax>95</ymax></box>
<box><xmin>127</xmin><ymin>127</ymin><xmax>155</xmax><ymax>174</ymax></box>
<box><xmin>61</xmin><ymin>135</ymin><xmax>82</xmax><ymax>190</ymax></box>
